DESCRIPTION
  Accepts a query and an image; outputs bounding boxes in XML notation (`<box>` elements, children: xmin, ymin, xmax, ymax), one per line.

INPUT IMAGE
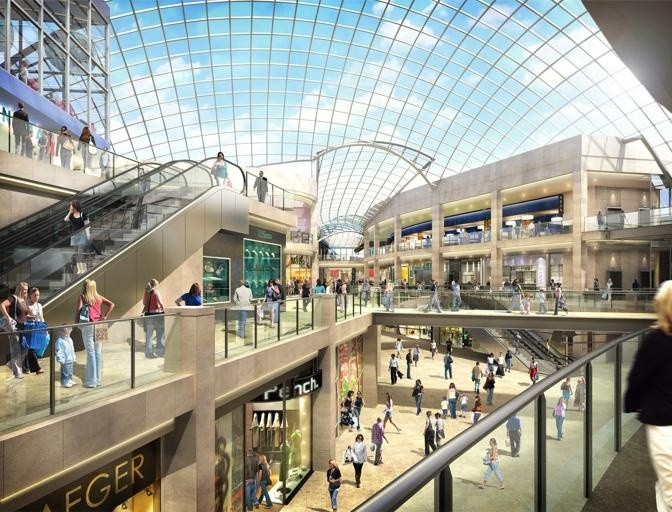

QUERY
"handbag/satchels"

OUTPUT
<box><xmin>138</xmin><ymin>314</ymin><xmax>144</xmax><ymax>327</ymax></box>
<box><xmin>272</xmin><ymin>291</ymin><xmax>281</xmax><ymax>301</ymax></box>
<box><xmin>436</xmin><ymin>429</ymin><xmax>445</xmax><ymax>442</ymax></box>
<box><xmin>35</xmin><ymin>334</ymin><xmax>50</xmax><ymax>356</ymax></box>
<box><xmin>78</xmin><ymin>304</ymin><xmax>89</xmax><ymax>330</ymax></box>
<box><xmin>425</xmin><ymin>428</ymin><xmax>434</xmax><ymax>440</ymax></box>
<box><xmin>0</xmin><ymin>316</ymin><xmax>17</xmax><ymax>334</ymax></box>
<box><xmin>446</xmin><ymin>355</ymin><xmax>453</xmax><ymax>363</ymax></box>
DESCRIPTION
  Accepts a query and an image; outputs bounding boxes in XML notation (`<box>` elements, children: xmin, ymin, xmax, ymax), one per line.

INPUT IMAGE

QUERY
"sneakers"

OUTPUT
<box><xmin>62</xmin><ymin>379</ymin><xmax>75</xmax><ymax>388</ymax></box>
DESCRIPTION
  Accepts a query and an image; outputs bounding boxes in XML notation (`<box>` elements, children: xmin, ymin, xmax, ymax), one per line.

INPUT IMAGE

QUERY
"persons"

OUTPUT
<box><xmin>10</xmin><ymin>100</ymin><xmax>111</xmax><ymax>178</ymax></box>
<box><xmin>596</xmin><ymin>210</ymin><xmax>603</xmax><ymax>229</ymax></box>
<box><xmin>210</xmin><ymin>152</ymin><xmax>228</xmax><ymax>186</ymax></box>
<box><xmin>53</xmin><ymin>323</ymin><xmax>78</xmax><ymax>387</ymax></box>
<box><xmin>619</xmin><ymin>280</ymin><xmax>671</xmax><ymax>512</ymax></box>
<box><xmin>75</xmin><ymin>278</ymin><xmax>116</xmax><ymax>389</ymax></box>
<box><xmin>63</xmin><ymin>199</ymin><xmax>92</xmax><ymax>275</ymax></box>
<box><xmin>606</xmin><ymin>278</ymin><xmax>613</xmax><ymax>290</ymax></box>
<box><xmin>552</xmin><ymin>283</ymin><xmax>568</xmax><ymax>314</ymax></box>
<box><xmin>19</xmin><ymin>61</ymin><xmax>29</xmax><ymax>85</ymax></box>
<box><xmin>593</xmin><ymin>278</ymin><xmax>600</xmax><ymax>290</ymax></box>
<box><xmin>538</xmin><ymin>288</ymin><xmax>547</xmax><ymax>314</ymax></box>
<box><xmin>631</xmin><ymin>278</ymin><xmax>640</xmax><ymax>291</ymax></box>
<box><xmin>23</xmin><ymin>286</ymin><xmax>46</xmax><ymax>374</ymax></box>
<box><xmin>252</xmin><ymin>171</ymin><xmax>268</xmax><ymax>204</ymax></box>
<box><xmin>548</xmin><ymin>279</ymin><xmax>556</xmax><ymax>290</ymax></box>
<box><xmin>141</xmin><ymin>278</ymin><xmax>167</xmax><ymax>358</ymax></box>
<box><xmin>425</xmin><ymin>236</ymin><xmax>431</xmax><ymax>243</ymax></box>
<box><xmin>342</xmin><ymin>334</ymin><xmax>587</xmax><ymax>492</ymax></box>
<box><xmin>1</xmin><ymin>281</ymin><xmax>32</xmax><ymax>379</ymax></box>
<box><xmin>256</xmin><ymin>277</ymin><xmax>535</xmax><ymax>328</ymax></box>
<box><xmin>232</xmin><ymin>279</ymin><xmax>254</xmax><ymax>339</ymax></box>
<box><xmin>174</xmin><ymin>283</ymin><xmax>202</xmax><ymax>307</ymax></box>
<box><xmin>326</xmin><ymin>459</ymin><xmax>342</xmax><ymax>511</ymax></box>
<box><xmin>254</xmin><ymin>455</ymin><xmax>273</xmax><ymax>508</ymax></box>
